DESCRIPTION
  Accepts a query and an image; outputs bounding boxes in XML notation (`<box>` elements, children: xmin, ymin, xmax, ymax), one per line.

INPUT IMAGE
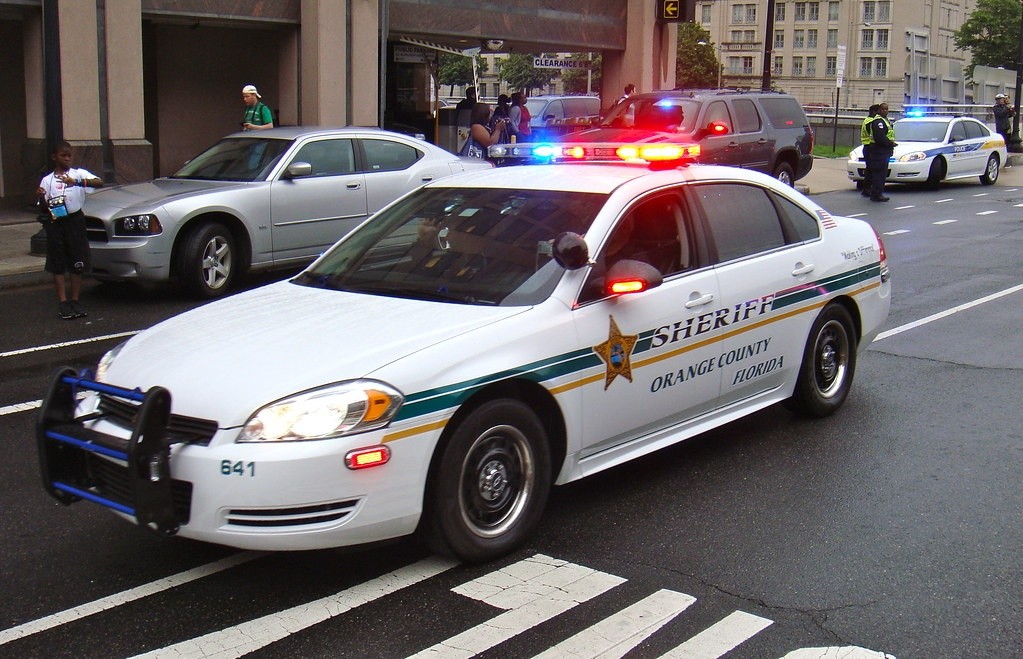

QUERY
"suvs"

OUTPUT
<box><xmin>488</xmin><ymin>86</ymin><xmax>815</xmax><ymax>189</ymax></box>
<box><xmin>509</xmin><ymin>94</ymin><xmax>601</xmax><ymax>128</ymax></box>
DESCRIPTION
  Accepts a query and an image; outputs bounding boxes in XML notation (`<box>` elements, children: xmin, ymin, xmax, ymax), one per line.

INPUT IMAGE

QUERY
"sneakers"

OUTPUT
<box><xmin>68</xmin><ymin>301</ymin><xmax>88</xmax><ymax>317</ymax></box>
<box><xmin>57</xmin><ymin>300</ymin><xmax>78</xmax><ymax>320</ymax></box>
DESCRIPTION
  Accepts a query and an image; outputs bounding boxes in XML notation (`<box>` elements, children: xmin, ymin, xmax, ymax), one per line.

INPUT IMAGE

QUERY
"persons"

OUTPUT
<box><xmin>869</xmin><ymin>103</ymin><xmax>898</xmax><ymax>201</ymax></box>
<box><xmin>548</xmin><ymin>205</ymin><xmax>641</xmax><ymax>299</ymax></box>
<box><xmin>456</xmin><ymin>87</ymin><xmax>532</xmax><ymax>166</ymax></box>
<box><xmin>36</xmin><ymin>140</ymin><xmax>104</xmax><ymax>319</ymax></box>
<box><xmin>242</xmin><ymin>84</ymin><xmax>273</xmax><ymax>172</ymax></box>
<box><xmin>862</xmin><ymin>104</ymin><xmax>879</xmax><ymax>197</ymax></box>
<box><xmin>617</xmin><ymin>84</ymin><xmax>636</xmax><ymax>126</ymax></box>
<box><xmin>993</xmin><ymin>93</ymin><xmax>1017</xmax><ymax>169</ymax></box>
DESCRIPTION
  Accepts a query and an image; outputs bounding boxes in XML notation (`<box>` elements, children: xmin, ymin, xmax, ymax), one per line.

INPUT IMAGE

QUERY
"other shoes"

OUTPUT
<box><xmin>862</xmin><ymin>190</ymin><xmax>872</xmax><ymax>198</ymax></box>
<box><xmin>870</xmin><ymin>192</ymin><xmax>889</xmax><ymax>203</ymax></box>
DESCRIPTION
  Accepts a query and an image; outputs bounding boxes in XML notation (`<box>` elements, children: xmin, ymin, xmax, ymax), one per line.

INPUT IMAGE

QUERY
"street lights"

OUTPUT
<box><xmin>698</xmin><ymin>42</ymin><xmax>721</xmax><ymax>91</ymax></box>
<box><xmin>846</xmin><ymin>22</ymin><xmax>870</xmax><ymax>108</ymax></box>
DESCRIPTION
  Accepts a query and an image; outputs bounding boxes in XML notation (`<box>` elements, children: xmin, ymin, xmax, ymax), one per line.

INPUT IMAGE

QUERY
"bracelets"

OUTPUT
<box><xmin>75</xmin><ymin>178</ymin><xmax>91</xmax><ymax>187</ymax></box>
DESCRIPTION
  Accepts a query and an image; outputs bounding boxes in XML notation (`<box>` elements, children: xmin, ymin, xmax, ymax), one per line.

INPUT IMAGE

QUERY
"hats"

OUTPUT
<box><xmin>995</xmin><ymin>94</ymin><xmax>1004</xmax><ymax>100</ymax></box>
<box><xmin>242</xmin><ymin>85</ymin><xmax>261</xmax><ymax>99</ymax></box>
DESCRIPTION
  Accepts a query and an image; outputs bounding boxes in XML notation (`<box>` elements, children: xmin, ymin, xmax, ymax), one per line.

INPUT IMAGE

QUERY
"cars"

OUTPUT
<box><xmin>35</xmin><ymin>141</ymin><xmax>893</xmax><ymax>569</ymax></box>
<box><xmin>81</xmin><ymin>126</ymin><xmax>497</xmax><ymax>298</ymax></box>
<box><xmin>848</xmin><ymin>111</ymin><xmax>1008</xmax><ymax>190</ymax></box>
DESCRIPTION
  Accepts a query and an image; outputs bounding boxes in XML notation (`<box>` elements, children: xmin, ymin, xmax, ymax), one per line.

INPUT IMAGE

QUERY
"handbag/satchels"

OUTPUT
<box><xmin>459</xmin><ymin>132</ymin><xmax>487</xmax><ymax>163</ymax></box>
<box><xmin>603</xmin><ymin>97</ymin><xmax>630</xmax><ymax>128</ymax></box>
